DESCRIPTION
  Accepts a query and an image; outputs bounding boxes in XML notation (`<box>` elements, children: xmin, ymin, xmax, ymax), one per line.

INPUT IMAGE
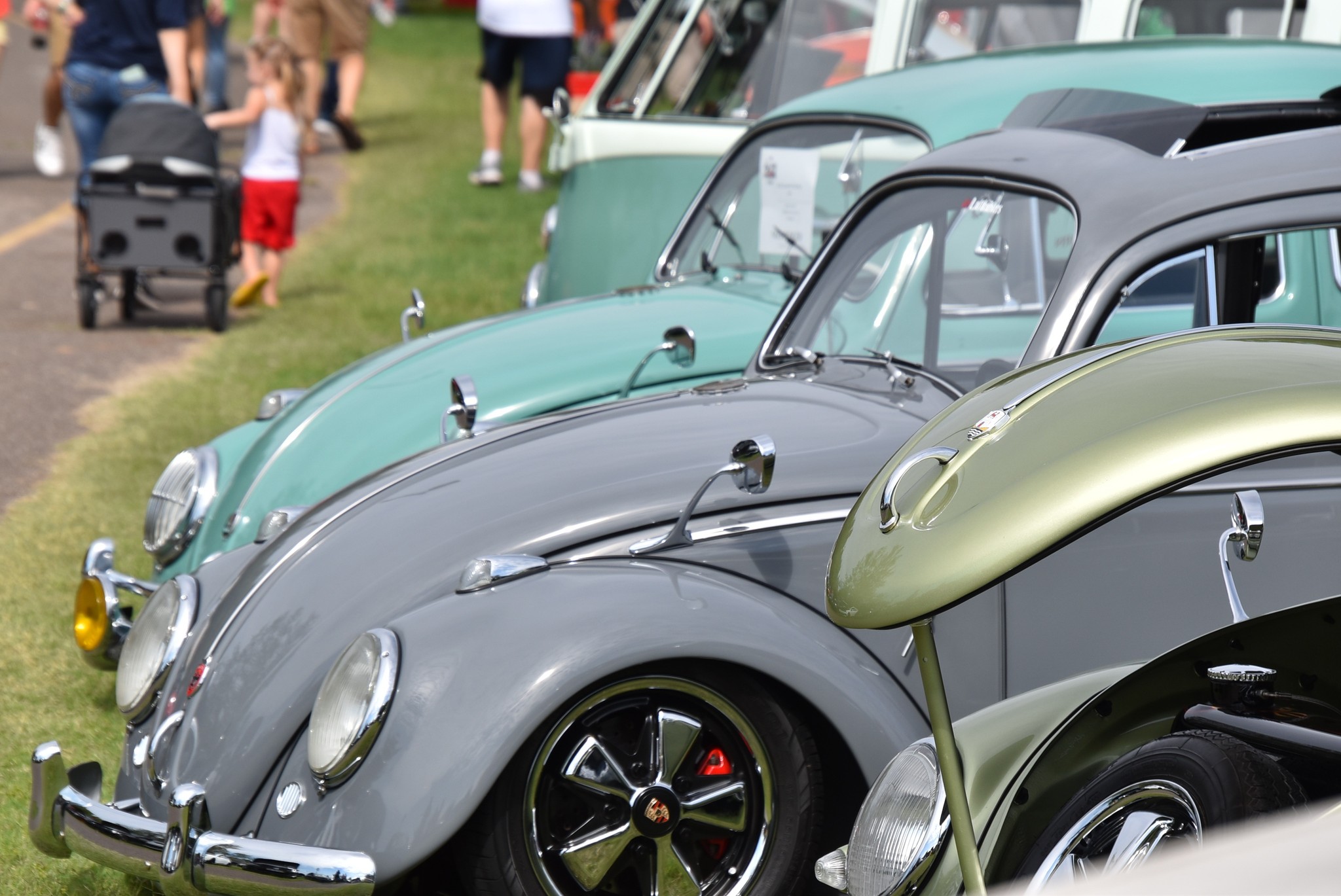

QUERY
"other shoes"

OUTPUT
<box><xmin>304</xmin><ymin>117</ymin><xmax>364</xmax><ymax>158</ymax></box>
<box><xmin>233</xmin><ymin>272</ymin><xmax>284</xmax><ymax>310</ymax></box>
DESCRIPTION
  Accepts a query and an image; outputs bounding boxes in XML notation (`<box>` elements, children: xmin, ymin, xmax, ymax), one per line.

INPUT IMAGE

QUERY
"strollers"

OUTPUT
<box><xmin>73</xmin><ymin>93</ymin><xmax>248</xmax><ymax>333</ymax></box>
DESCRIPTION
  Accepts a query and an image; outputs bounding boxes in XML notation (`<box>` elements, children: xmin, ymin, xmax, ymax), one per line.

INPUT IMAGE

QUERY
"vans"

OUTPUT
<box><xmin>519</xmin><ymin>0</ymin><xmax>1341</xmax><ymax>318</ymax></box>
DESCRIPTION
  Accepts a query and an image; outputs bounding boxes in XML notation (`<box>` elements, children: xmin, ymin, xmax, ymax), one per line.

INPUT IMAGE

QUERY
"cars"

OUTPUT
<box><xmin>72</xmin><ymin>35</ymin><xmax>1341</xmax><ymax>667</ymax></box>
<box><xmin>813</xmin><ymin>322</ymin><xmax>1341</xmax><ymax>896</ymax></box>
<box><xmin>26</xmin><ymin>88</ymin><xmax>1341</xmax><ymax>896</ymax></box>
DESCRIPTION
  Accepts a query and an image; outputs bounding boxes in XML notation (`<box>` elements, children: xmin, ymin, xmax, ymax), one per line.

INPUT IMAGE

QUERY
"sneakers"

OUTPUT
<box><xmin>466</xmin><ymin>154</ymin><xmax>544</xmax><ymax>192</ymax></box>
<box><xmin>30</xmin><ymin>121</ymin><xmax>68</xmax><ymax>175</ymax></box>
<box><xmin>73</xmin><ymin>273</ymin><xmax>164</xmax><ymax>313</ymax></box>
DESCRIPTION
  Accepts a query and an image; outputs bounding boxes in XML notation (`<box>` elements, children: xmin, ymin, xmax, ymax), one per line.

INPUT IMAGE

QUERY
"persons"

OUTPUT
<box><xmin>21</xmin><ymin>0</ymin><xmax>83</xmax><ymax>178</ymax></box>
<box><xmin>278</xmin><ymin>1</ymin><xmax>396</xmax><ymax>157</ymax></box>
<box><xmin>203</xmin><ymin>38</ymin><xmax>320</xmax><ymax>309</ymax></box>
<box><xmin>468</xmin><ymin>0</ymin><xmax>575</xmax><ymax>190</ymax></box>
<box><xmin>61</xmin><ymin>0</ymin><xmax>192</xmax><ymax>329</ymax></box>
